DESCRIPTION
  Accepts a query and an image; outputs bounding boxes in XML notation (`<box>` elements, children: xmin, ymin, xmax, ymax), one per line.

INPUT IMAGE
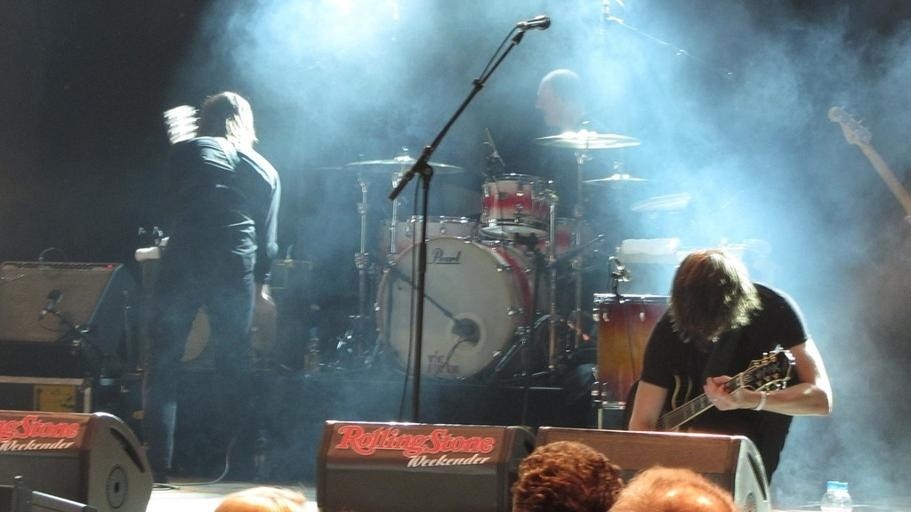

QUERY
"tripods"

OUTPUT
<box><xmin>564</xmin><ymin>161</ymin><xmax>593</xmax><ymax>352</ymax></box>
<box><xmin>330</xmin><ymin>174</ymin><xmax>375</xmax><ymax>370</ymax></box>
<box><xmin>494</xmin><ymin>205</ymin><xmax>587</xmax><ymax>385</ymax></box>
<box><xmin>366</xmin><ymin>179</ymin><xmax>404</xmax><ymax>373</ymax></box>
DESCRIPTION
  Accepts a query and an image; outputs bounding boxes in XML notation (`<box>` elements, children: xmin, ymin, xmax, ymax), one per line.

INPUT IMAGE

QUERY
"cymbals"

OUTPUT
<box><xmin>536</xmin><ymin>132</ymin><xmax>640</xmax><ymax>149</ymax></box>
<box><xmin>348</xmin><ymin>158</ymin><xmax>463</xmax><ymax>176</ymax></box>
<box><xmin>584</xmin><ymin>176</ymin><xmax>645</xmax><ymax>183</ymax></box>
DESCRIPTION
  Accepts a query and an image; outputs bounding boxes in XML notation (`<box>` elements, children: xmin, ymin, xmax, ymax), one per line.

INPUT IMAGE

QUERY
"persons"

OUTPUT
<box><xmin>536</xmin><ymin>63</ymin><xmax>606</xmax><ymax>152</ymax></box>
<box><xmin>512</xmin><ymin>441</ymin><xmax>620</xmax><ymax>512</ymax></box>
<box><xmin>624</xmin><ymin>251</ymin><xmax>832</xmax><ymax>487</ymax></box>
<box><xmin>141</xmin><ymin>92</ymin><xmax>279</xmax><ymax>486</ymax></box>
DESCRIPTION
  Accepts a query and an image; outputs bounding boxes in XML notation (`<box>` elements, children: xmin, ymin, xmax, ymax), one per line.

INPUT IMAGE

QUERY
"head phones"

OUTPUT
<box><xmin>607</xmin><ymin>256</ymin><xmax>634</xmax><ymax>285</ymax></box>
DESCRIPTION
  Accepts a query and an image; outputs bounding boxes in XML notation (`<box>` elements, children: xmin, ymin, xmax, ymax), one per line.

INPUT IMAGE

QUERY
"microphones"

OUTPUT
<box><xmin>38</xmin><ymin>289</ymin><xmax>62</xmax><ymax>320</ymax></box>
<box><xmin>515</xmin><ymin>15</ymin><xmax>554</xmax><ymax>34</ymax></box>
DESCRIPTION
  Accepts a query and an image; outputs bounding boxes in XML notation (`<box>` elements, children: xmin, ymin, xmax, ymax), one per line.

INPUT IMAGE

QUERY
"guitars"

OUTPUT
<box><xmin>622</xmin><ymin>345</ymin><xmax>795</xmax><ymax>431</ymax></box>
<box><xmin>828</xmin><ymin>107</ymin><xmax>911</xmax><ymax>218</ymax></box>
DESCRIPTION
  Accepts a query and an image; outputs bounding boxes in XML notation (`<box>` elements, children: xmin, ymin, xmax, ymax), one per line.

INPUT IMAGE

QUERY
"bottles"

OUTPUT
<box><xmin>821</xmin><ymin>481</ymin><xmax>856</xmax><ymax>511</ymax></box>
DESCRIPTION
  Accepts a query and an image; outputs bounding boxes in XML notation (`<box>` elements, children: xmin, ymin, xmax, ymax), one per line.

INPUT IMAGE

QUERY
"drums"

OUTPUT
<box><xmin>482</xmin><ymin>174</ymin><xmax>552</xmax><ymax>238</ymax></box>
<box><xmin>376</xmin><ymin>237</ymin><xmax>533</xmax><ymax>382</ymax></box>
<box><xmin>592</xmin><ymin>294</ymin><xmax>672</xmax><ymax>412</ymax></box>
<box><xmin>379</xmin><ymin>218</ymin><xmax>477</xmax><ymax>261</ymax></box>
<box><xmin>537</xmin><ymin>219</ymin><xmax>597</xmax><ymax>267</ymax></box>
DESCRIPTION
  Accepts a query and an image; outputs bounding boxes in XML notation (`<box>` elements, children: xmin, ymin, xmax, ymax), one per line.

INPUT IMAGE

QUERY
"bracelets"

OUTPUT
<box><xmin>755</xmin><ymin>389</ymin><xmax>765</xmax><ymax>412</ymax></box>
